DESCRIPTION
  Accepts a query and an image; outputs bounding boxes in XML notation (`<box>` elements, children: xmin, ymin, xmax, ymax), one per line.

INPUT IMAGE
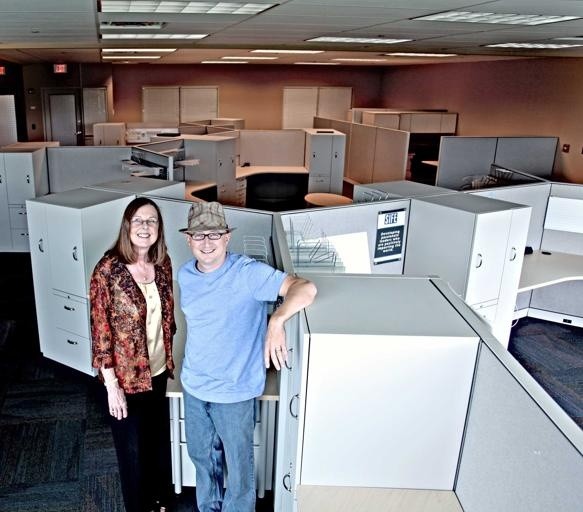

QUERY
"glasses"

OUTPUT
<box><xmin>187</xmin><ymin>232</ymin><xmax>229</xmax><ymax>240</ymax></box>
<box><xmin>132</xmin><ymin>218</ymin><xmax>158</xmax><ymax>225</ymax></box>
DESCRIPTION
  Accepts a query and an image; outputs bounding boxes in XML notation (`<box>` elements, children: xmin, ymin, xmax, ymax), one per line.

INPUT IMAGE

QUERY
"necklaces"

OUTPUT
<box><xmin>142</xmin><ymin>275</ymin><xmax>148</xmax><ymax>281</ymax></box>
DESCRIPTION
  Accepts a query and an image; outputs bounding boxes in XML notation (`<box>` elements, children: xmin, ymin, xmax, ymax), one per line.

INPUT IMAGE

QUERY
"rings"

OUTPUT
<box><xmin>111</xmin><ymin>410</ymin><xmax>118</xmax><ymax>413</ymax></box>
<box><xmin>275</xmin><ymin>346</ymin><xmax>282</xmax><ymax>351</ymax></box>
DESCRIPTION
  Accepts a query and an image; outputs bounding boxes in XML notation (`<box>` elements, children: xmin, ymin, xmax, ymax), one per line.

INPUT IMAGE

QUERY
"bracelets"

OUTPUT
<box><xmin>103</xmin><ymin>376</ymin><xmax>118</xmax><ymax>386</ymax></box>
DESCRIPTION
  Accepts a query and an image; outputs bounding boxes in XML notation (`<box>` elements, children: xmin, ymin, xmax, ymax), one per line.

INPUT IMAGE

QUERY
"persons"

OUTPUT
<box><xmin>89</xmin><ymin>196</ymin><xmax>177</xmax><ymax>512</ymax></box>
<box><xmin>178</xmin><ymin>201</ymin><xmax>318</xmax><ymax>512</ymax></box>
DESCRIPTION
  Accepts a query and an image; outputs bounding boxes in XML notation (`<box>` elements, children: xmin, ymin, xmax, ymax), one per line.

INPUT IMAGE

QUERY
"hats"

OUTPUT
<box><xmin>178</xmin><ymin>202</ymin><xmax>236</xmax><ymax>234</ymax></box>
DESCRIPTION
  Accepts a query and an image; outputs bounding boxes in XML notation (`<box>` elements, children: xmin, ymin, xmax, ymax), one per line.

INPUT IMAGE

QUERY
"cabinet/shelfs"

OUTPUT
<box><xmin>164</xmin><ymin>79</ymin><xmax>583</xmax><ymax>512</ymax></box>
<box><xmin>1</xmin><ymin>79</ymin><xmax>184</xmax><ymax>377</ymax></box>
